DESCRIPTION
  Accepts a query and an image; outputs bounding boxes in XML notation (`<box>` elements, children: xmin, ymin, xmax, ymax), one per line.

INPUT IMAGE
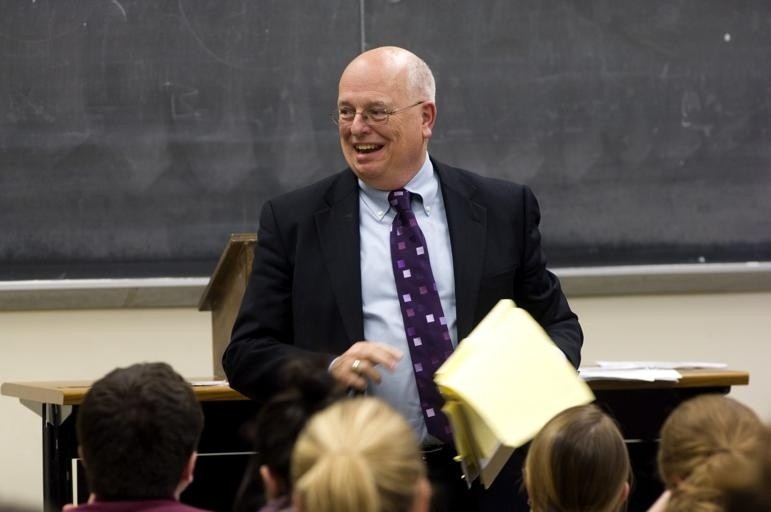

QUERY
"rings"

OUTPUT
<box><xmin>351</xmin><ymin>360</ymin><xmax>360</xmax><ymax>372</ymax></box>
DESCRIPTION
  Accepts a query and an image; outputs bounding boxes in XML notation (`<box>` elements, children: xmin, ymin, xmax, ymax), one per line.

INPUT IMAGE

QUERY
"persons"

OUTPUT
<box><xmin>669</xmin><ymin>426</ymin><xmax>771</xmax><ymax>512</ymax></box>
<box><xmin>656</xmin><ymin>394</ymin><xmax>761</xmax><ymax>492</ymax></box>
<box><xmin>290</xmin><ymin>396</ymin><xmax>434</xmax><ymax>512</ymax></box>
<box><xmin>221</xmin><ymin>46</ymin><xmax>584</xmax><ymax>512</ymax></box>
<box><xmin>523</xmin><ymin>406</ymin><xmax>634</xmax><ymax>512</ymax></box>
<box><xmin>62</xmin><ymin>362</ymin><xmax>215</xmax><ymax>512</ymax></box>
<box><xmin>235</xmin><ymin>357</ymin><xmax>346</xmax><ymax>512</ymax></box>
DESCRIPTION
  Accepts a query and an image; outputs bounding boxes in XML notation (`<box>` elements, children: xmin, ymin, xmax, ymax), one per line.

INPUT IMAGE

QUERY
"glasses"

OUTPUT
<box><xmin>331</xmin><ymin>101</ymin><xmax>426</xmax><ymax>127</ymax></box>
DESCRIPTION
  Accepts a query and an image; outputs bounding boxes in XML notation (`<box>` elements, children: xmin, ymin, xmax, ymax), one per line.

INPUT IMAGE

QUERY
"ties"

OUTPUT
<box><xmin>389</xmin><ymin>188</ymin><xmax>460</xmax><ymax>444</ymax></box>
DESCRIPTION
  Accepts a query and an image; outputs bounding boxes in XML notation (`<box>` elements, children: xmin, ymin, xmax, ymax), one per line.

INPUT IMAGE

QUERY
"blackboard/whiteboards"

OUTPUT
<box><xmin>0</xmin><ymin>0</ymin><xmax>771</xmax><ymax>292</ymax></box>
